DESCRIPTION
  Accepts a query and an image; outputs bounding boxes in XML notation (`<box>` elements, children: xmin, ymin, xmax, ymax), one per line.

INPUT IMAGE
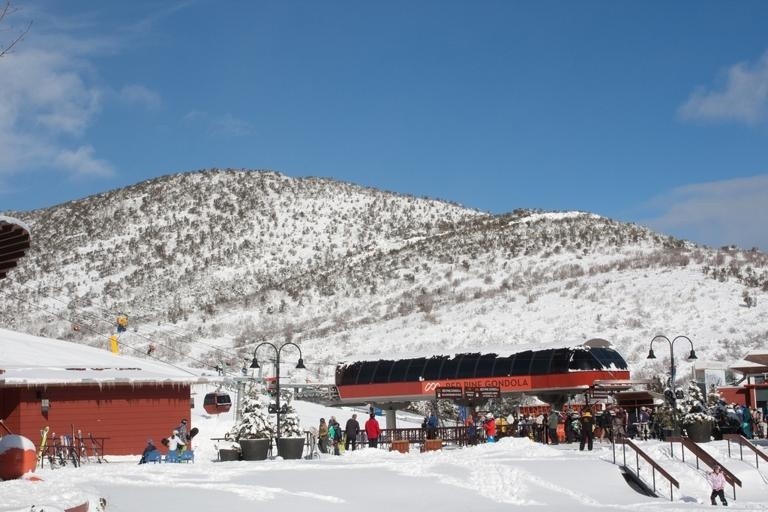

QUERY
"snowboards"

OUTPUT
<box><xmin>185</xmin><ymin>427</ymin><xmax>199</xmax><ymax>441</ymax></box>
<box><xmin>161</xmin><ymin>438</ymin><xmax>183</xmax><ymax>450</ymax></box>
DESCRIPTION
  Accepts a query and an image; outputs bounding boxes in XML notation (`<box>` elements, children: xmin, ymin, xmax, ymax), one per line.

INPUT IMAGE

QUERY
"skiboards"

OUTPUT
<box><xmin>36</xmin><ymin>426</ymin><xmax>102</xmax><ymax>468</ymax></box>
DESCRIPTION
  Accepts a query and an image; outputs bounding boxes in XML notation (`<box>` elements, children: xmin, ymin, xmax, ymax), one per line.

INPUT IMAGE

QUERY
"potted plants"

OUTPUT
<box><xmin>684</xmin><ymin>414</ymin><xmax>713</xmax><ymax>443</ymax></box>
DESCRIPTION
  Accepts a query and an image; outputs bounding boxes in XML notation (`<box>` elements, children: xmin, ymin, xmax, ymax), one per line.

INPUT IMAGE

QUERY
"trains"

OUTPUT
<box><xmin>334</xmin><ymin>347</ymin><xmax>631</xmax><ymax>401</ymax></box>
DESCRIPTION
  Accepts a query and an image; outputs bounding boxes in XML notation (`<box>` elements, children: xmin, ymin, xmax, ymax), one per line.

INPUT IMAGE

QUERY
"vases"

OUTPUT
<box><xmin>217</xmin><ymin>438</ymin><xmax>305</xmax><ymax>461</ymax></box>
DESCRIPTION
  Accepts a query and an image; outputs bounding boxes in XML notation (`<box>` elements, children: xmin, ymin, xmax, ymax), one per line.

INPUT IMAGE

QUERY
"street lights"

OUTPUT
<box><xmin>647</xmin><ymin>335</ymin><xmax>698</xmax><ymax>408</ymax></box>
<box><xmin>250</xmin><ymin>342</ymin><xmax>307</xmax><ymax>436</ymax></box>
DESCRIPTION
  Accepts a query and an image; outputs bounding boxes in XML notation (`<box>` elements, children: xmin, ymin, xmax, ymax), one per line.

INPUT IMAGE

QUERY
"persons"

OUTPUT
<box><xmin>138</xmin><ymin>419</ymin><xmax>189</xmax><ymax>466</ymax></box>
<box><xmin>465</xmin><ymin>406</ymin><xmax>653</xmax><ymax>450</ymax></box>
<box><xmin>318</xmin><ymin>413</ymin><xmax>380</xmax><ymax>455</ymax></box>
<box><xmin>424</xmin><ymin>409</ymin><xmax>438</xmax><ymax>440</ymax></box>
<box><xmin>711</xmin><ymin>465</ymin><xmax>730</xmax><ymax>505</ymax></box>
